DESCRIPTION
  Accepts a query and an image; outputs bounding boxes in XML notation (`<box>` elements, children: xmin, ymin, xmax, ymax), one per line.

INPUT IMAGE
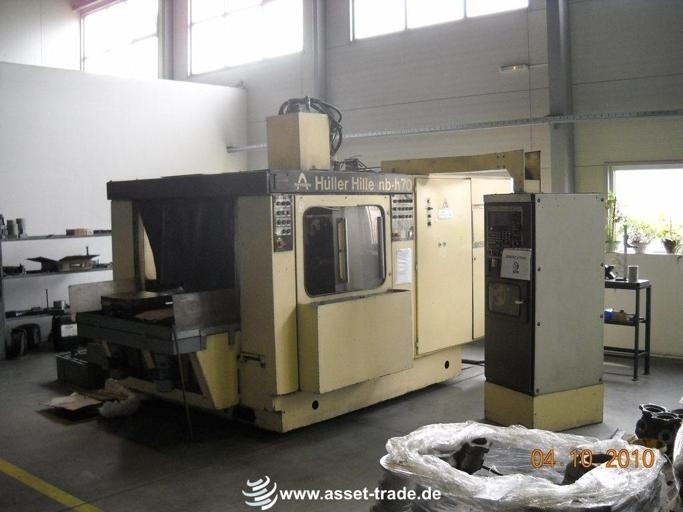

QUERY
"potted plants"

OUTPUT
<box><xmin>606</xmin><ymin>193</ymin><xmax>683</xmax><ymax>254</ymax></box>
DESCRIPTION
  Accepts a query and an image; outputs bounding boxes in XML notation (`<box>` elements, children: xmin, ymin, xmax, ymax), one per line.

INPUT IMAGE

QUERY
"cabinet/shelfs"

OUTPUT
<box><xmin>0</xmin><ymin>230</ymin><xmax>113</xmax><ymax>358</ymax></box>
<box><xmin>605</xmin><ymin>277</ymin><xmax>651</xmax><ymax>381</ymax></box>
